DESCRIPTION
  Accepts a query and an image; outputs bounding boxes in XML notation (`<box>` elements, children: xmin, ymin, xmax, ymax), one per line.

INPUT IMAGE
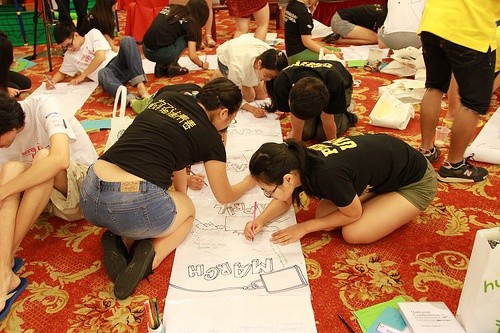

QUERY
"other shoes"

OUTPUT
<box><xmin>132</xmin><ymin>97</ymin><xmax>149</xmax><ymax>114</ymax></box>
<box><xmin>155</xmin><ymin>65</ymin><xmax>167</xmax><ymax>76</ymax></box>
<box><xmin>322</xmin><ymin>33</ymin><xmax>340</xmax><ymax>43</ymax></box>
<box><xmin>168</xmin><ymin>62</ymin><xmax>189</xmax><ymax>76</ymax></box>
<box><xmin>102</xmin><ymin>230</ymin><xmax>128</xmax><ymax>281</ymax></box>
<box><xmin>344</xmin><ymin>111</ymin><xmax>358</xmax><ymax>126</ymax></box>
<box><xmin>204</xmin><ymin>34</ymin><xmax>216</xmax><ymax>47</ymax></box>
<box><xmin>115</xmin><ymin>240</ymin><xmax>156</xmax><ymax>298</ymax></box>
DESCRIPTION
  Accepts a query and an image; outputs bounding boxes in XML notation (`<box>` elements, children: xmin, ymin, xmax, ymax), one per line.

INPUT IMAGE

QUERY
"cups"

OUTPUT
<box><xmin>434</xmin><ymin>126</ymin><xmax>451</xmax><ymax>147</ymax></box>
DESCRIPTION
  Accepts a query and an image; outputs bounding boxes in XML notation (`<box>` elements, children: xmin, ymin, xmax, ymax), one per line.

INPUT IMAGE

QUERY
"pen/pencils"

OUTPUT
<box><xmin>205</xmin><ymin>54</ymin><xmax>207</xmax><ymax>62</ymax></box>
<box><xmin>250</xmin><ymin>96</ymin><xmax>267</xmax><ymax>118</ymax></box>
<box><xmin>18</xmin><ymin>89</ymin><xmax>32</xmax><ymax>92</ymax></box>
<box><xmin>187</xmin><ymin>168</ymin><xmax>208</xmax><ymax>186</ymax></box>
<box><xmin>338</xmin><ymin>314</ymin><xmax>356</xmax><ymax>333</ymax></box>
<box><xmin>45</xmin><ymin>72</ymin><xmax>55</xmax><ymax>89</ymax></box>
<box><xmin>252</xmin><ymin>202</ymin><xmax>257</xmax><ymax>241</ymax></box>
<box><xmin>441</xmin><ymin>119</ymin><xmax>443</xmax><ymax>130</ymax></box>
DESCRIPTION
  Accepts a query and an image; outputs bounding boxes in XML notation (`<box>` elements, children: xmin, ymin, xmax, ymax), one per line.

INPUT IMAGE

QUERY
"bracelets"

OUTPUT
<box><xmin>199</xmin><ymin>61</ymin><xmax>203</xmax><ymax>67</ymax></box>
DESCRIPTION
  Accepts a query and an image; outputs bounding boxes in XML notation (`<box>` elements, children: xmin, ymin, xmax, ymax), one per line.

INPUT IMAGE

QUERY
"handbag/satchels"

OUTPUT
<box><xmin>101</xmin><ymin>86</ymin><xmax>134</xmax><ymax>154</ymax></box>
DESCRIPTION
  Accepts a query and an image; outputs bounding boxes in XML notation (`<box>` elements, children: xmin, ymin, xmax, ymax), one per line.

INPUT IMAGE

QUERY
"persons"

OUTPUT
<box><xmin>243</xmin><ymin>133</ymin><xmax>438</xmax><ymax>245</ymax></box>
<box><xmin>53</xmin><ymin>0</ymin><xmax>216</xmax><ymax>54</ymax></box>
<box><xmin>284</xmin><ymin>0</ymin><xmax>344</xmax><ymax>67</ymax></box>
<box><xmin>321</xmin><ymin>4</ymin><xmax>388</xmax><ymax>45</ymax></box>
<box><xmin>215</xmin><ymin>34</ymin><xmax>289</xmax><ymax>118</ymax></box>
<box><xmin>142</xmin><ymin>0</ymin><xmax>209</xmax><ymax>79</ymax></box>
<box><xmin>80</xmin><ymin>77</ymin><xmax>257</xmax><ymax>300</ymax></box>
<box><xmin>227</xmin><ymin>0</ymin><xmax>270</xmax><ymax>42</ymax></box>
<box><xmin>273</xmin><ymin>60</ymin><xmax>358</xmax><ymax>145</ymax></box>
<box><xmin>45</xmin><ymin>20</ymin><xmax>151</xmax><ymax>115</ymax></box>
<box><xmin>377</xmin><ymin>0</ymin><xmax>425</xmax><ymax>51</ymax></box>
<box><xmin>0</xmin><ymin>93</ymin><xmax>100</xmax><ymax>322</ymax></box>
<box><xmin>0</xmin><ymin>31</ymin><xmax>31</xmax><ymax>98</ymax></box>
<box><xmin>417</xmin><ymin>0</ymin><xmax>500</xmax><ymax>182</ymax></box>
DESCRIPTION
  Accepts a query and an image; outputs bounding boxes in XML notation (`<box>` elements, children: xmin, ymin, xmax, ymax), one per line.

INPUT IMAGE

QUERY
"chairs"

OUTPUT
<box><xmin>125</xmin><ymin>0</ymin><xmax>170</xmax><ymax>45</ymax></box>
<box><xmin>116</xmin><ymin>0</ymin><xmax>136</xmax><ymax>11</ymax></box>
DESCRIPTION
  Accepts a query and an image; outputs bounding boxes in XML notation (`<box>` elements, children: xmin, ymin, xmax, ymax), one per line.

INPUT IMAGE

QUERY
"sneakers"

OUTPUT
<box><xmin>417</xmin><ymin>144</ymin><xmax>441</xmax><ymax>162</ymax></box>
<box><xmin>437</xmin><ymin>152</ymin><xmax>488</xmax><ymax>182</ymax></box>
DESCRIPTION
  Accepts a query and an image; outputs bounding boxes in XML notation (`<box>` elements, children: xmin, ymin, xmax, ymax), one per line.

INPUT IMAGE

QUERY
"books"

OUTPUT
<box><xmin>353</xmin><ymin>296</ymin><xmax>467</xmax><ymax>333</ymax></box>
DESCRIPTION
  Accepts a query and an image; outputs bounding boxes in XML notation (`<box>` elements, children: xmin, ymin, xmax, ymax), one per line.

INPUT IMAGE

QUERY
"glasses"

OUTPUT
<box><xmin>61</xmin><ymin>33</ymin><xmax>74</xmax><ymax>49</ymax></box>
<box><xmin>219</xmin><ymin>107</ymin><xmax>238</xmax><ymax>127</ymax></box>
<box><xmin>262</xmin><ymin>179</ymin><xmax>282</xmax><ymax>199</ymax></box>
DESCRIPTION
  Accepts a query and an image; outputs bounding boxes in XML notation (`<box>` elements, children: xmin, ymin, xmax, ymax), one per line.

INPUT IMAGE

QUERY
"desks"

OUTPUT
<box><xmin>211</xmin><ymin>1</ymin><xmax>280</xmax><ymax>41</ymax></box>
<box><xmin>312</xmin><ymin>0</ymin><xmax>388</xmax><ymax>28</ymax></box>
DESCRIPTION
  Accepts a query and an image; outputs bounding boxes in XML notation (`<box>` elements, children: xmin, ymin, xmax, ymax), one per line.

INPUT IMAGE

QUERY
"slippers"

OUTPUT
<box><xmin>11</xmin><ymin>256</ymin><xmax>24</xmax><ymax>273</ymax></box>
<box><xmin>0</xmin><ymin>277</ymin><xmax>28</xmax><ymax>320</ymax></box>
